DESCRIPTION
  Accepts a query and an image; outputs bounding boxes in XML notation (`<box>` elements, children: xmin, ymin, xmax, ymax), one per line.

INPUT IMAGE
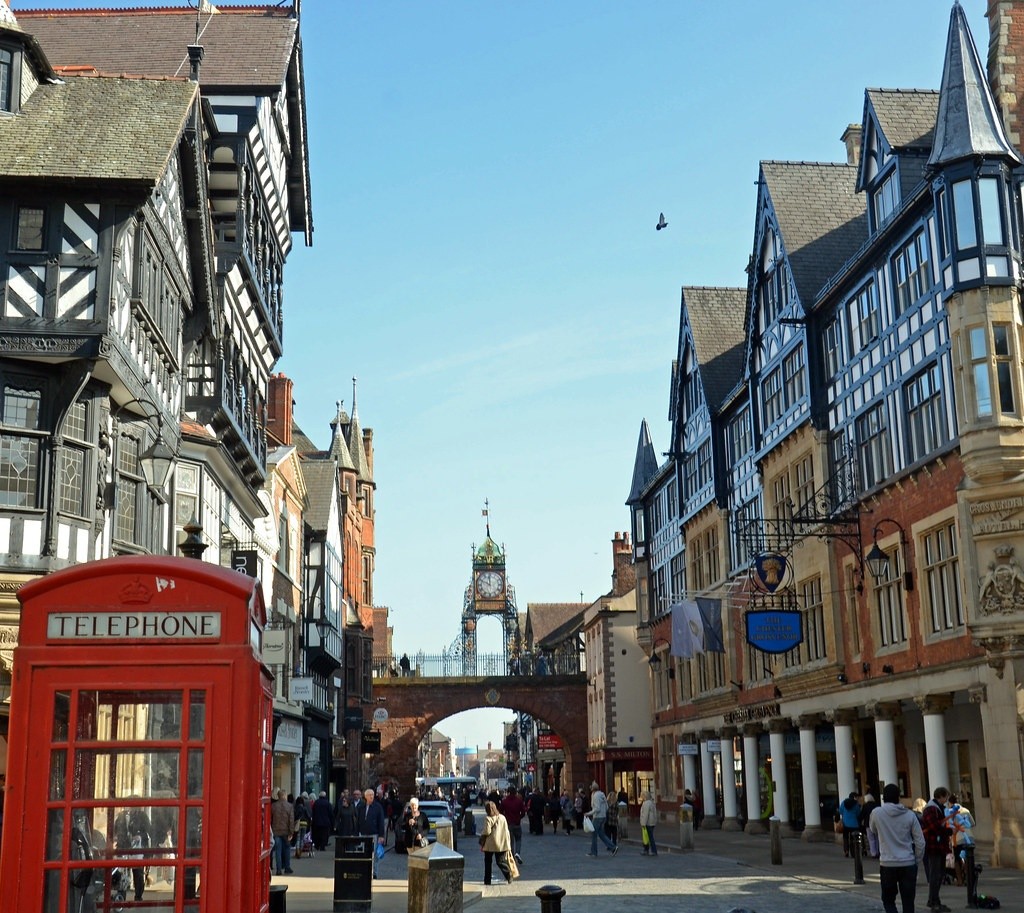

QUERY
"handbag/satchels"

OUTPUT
<box><xmin>642</xmin><ymin>826</ymin><xmax>649</xmax><ymax>845</ymax></box>
<box><xmin>506</xmin><ymin>851</ymin><xmax>520</xmax><ymax>879</ymax></box>
<box><xmin>421</xmin><ymin>837</ymin><xmax>429</xmax><ymax>847</ymax></box>
<box><xmin>376</xmin><ymin>843</ymin><xmax>385</xmax><ymax>859</ymax></box>
<box><xmin>583</xmin><ymin>813</ymin><xmax>596</xmax><ymax>833</ymax></box>
<box><xmin>295</xmin><ymin>819</ymin><xmax>300</xmax><ymax>832</ymax></box>
<box><xmin>523</xmin><ymin>800</ymin><xmax>531</xmax><ymax>811</ymax></box>
<box><xmin>833</xmin><ymin>812</ymin><xmax>843</xmax><ymax>833</ymax></box>
<box><xmin>479</xmin><ymin>835</ymin><xmax>487</xmax><ymax>846</ymax></box>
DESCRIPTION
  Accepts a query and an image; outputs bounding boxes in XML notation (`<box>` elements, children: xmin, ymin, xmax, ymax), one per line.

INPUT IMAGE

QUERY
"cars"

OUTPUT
<box><xmin>394</xmin><ymin>800</ymin><xmax>460</xmax><ymax>853</ymax></box>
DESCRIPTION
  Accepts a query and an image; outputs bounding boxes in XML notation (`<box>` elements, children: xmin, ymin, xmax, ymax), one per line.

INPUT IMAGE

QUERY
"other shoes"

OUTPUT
<box><xmin>650</xmin><ymin>851</ymin><xmax>657</xmax><ymax>856</ymax></box>
<box><xmin>845</xmin><ymin>852</ymin><xmax>849</xmax><ymax>857</ymax></box>
<box><xmin>964</xmin><ymin>880</ymin><xmax>968</xmax><ymax>885</ymax></box>
<box><xmin>508</xmin><ymin>878</ymin><xmax>514</xmax><ymax>884</ymax></box>
<box><xmin>285</xmin><ymin>868</ymin><xmax>294</xmax><ymax>873</ymax></box>
<box><xmin>135</xmin><ymin>898</ymin><xmax>143</xmax><ymax>901</ymax></box>
<box><xmin>932</xmin><ymin>905</ymin><xmax>951</xmax><ymax>913</ymax></box>
<box><xmin>586</xmin><ymin>853</ymin><xmax>596</xmax><ymax>857</ymax></box>
<box><xmin>640</xmin><ymin>851</ymin><xmax>648</xmax><ymax>855</ymax></box>
<box><xmin>872</xmin><ymin>855</ymin><xmax>880</xmax><ymax>859</ymax></box>
<box><xmin>565</xmin><ymin>833</ymin><xmax>569</xmax><ymax>835</ymax></box>
<box><xmin>957</xmin><ymin>880</ymin><xmax>963</xmax><ymax>886</ymax></box>
<box><xmin>612</xmin><ymin>846</ymin><xmax>618</xmax><ymax>856</ymax></box>
<box><xmin>276</xmin><ymin>871</ymin><xmax>282</xmax><ymax>875</ymax></box>
<box><xmin>851</xmin><ymin>854</ymin><xmax>855</xmax><ymax>858</ymax></box>
<box><xmin>515</xmin><ymin>853</ymin><xmax>522</xmax><ymax>864</ymax></box>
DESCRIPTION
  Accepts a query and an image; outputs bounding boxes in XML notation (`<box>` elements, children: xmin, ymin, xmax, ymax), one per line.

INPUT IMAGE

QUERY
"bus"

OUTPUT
<box><xmin>414</xmin><ymin>776</ymin><xmax>480</xmax><ymax>808</ymax></box>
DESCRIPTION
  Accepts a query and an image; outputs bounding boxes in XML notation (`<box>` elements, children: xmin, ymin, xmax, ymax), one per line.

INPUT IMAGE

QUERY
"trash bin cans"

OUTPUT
<box><xmin>333</xmin><ymin>836</ymin><xmax>375</xmax><ymax>913</ymax></box>
<box><xmin>407</xmin><ymin>842</ymin><xmax>465</xmax><ymax>913</ymax></box>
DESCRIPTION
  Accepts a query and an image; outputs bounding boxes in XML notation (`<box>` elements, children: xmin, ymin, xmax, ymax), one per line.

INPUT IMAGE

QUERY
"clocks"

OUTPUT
<box><xmin>477</xmin><ymin>571</ymin><xmax>504</xmax><ymax>599</ymax></box>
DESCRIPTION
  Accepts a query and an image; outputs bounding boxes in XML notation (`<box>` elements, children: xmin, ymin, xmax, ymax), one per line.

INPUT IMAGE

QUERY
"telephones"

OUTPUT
<box><xmin>51</xmin><ymin>809</ymin><xmax>96</xmax><ymax>913</ymax></box>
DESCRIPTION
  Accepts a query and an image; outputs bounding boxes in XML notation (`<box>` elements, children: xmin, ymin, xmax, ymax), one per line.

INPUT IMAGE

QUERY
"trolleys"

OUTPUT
<box><xmin>294</xmin><ymin>829</ymin><xmax>314</xmax><ymax>858</ymax></box>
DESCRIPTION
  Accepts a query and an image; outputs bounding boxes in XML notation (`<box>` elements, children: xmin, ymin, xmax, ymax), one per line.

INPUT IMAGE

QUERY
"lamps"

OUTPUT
<box><xmin>299</xmin><ymin>595</ymin><xmax>332</xmax><ymax>638</ymax></box>
<box><xmin>649</xmin><ymin>638</ymin><xmax>675</xmax><ymax>678</ymax></box>
<box><xmin>866</xmin><ymin>519</ymin><xmax>913</xmax><ymax>592</ymax></box>
<box><xmin>101</xmin><ymin>399</ymin><xmax>176</xmax><ymax>494</ymax></box>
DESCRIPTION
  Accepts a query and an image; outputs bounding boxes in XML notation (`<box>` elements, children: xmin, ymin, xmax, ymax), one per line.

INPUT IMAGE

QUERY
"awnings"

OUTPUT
<box><xmin>416</xmin><ymin>776</ymin><xmax>477</xmax><ymax>785</ymax></box>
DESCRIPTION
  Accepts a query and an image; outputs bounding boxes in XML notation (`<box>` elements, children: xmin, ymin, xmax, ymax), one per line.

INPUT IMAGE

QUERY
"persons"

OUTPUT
<box><xmin>919</xmin><ymin>787</ymin><xmax>958</xmax><ymax>913</ymax></box>
<box><xmin>684</xmin><ymin>789</ymin><xmax>696</xmax><ymax>829</ymax></box>
<box><xmin>271</xmin><ymin>785</ymin><xmax>333</xmax><ymax>876</ymax></box>
<box><xmin>457</xmin><ymin>786</ymin><xmax>628</xmax><ymax>837</ymax></box>
<box><xmin>870</xmin><ymin>783</ymin><xmax>925</xmax><ymax>913</ymax></box>
<box><xmin>334</xmin><ymin>787</ymin><xmax>402</xmax><ymax>846</ymax></box>
<box><xmin>480</xmin><ymin>802</ymin><xmax>514</xmax><ymax>885</ymax></box>
<box><xmin>509</xmin><ymin>653</ymin><xmax>521</xmax><ymax>675</ymax></box>
<box><xmin>838</xmin><ymin>791</ymin><xmax>976</xmax><ymax>886</ymax></box>
<box><xmin>640</xmin><ymin>791</ymin><xmax>657</xmax><ymax>855</ymax></box>
<box><xmin>583</xmin><ymin>783</ymin><xmax>619</xmax><ymax>857</ymax></box>
<box><xmin>403</xmin><ymin>798</ymin><xmax>430</xmax><ymax>854</ymax></box>
<box><xmin>359</xmin><ymin>789</ymin><xmax>384</xmax><ymax>879</ymax></box>
<box><xmin>113</xmin><ymin>797</ymin><xmax>149</xmax><ymax>903</ymax></box>
<box><xmin>385</xmin><ymin>653</ymin><xmax>410</xmax><ymax>677</ymax></box>
<box><xmin>498</xmin><ymin>787</ymin><xmax>525</xmax><ymax>864</ymax></box>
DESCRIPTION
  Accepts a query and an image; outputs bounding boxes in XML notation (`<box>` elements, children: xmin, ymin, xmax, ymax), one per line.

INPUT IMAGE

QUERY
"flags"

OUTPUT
<box><xmin>669</xmin><ymin>597</ymin><xmax>726</xmax><ymax>657</ymax></box>
